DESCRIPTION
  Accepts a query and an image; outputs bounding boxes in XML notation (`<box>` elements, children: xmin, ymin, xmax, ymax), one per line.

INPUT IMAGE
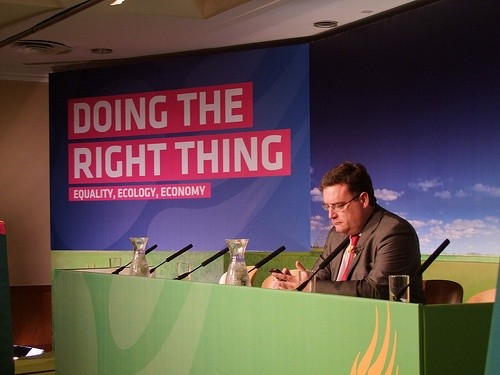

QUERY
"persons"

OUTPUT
<box><xmin>272</xmin><ymin>160</ymin><xmax>425</xmax><ymax>304</ymax></box>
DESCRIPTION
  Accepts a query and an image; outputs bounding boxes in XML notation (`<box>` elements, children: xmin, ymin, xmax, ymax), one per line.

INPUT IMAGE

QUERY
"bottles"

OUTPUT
<box><xmin>130</xmin><ymin>237</ymin><xmax>150</xmax><ymax>277</ymax></box>
<box><xmin>226</xmin><ymin>239</ymin><xmax>252</xmax><ymax>287</ymax></box>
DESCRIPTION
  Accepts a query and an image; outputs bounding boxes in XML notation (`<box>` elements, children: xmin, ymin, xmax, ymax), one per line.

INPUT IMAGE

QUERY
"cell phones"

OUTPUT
<box><xmin>269</xmin><ymin>268</ymin><xmax>285</xmax><ymax>282</ymax></box>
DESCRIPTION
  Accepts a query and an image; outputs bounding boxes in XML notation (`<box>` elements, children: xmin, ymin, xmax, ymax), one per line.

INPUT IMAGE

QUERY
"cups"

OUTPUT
<box><xmin>299</xmin><ymin>271</ymin><xmax>317</xmax><ymax>293</ymax></box>
<box><xmin>389</xmin><ymin>275</ymin><xmax>410</xmax><ymax>302</ymax></box>
<box><xmin>177</xmin><ymin>263</ymin><xmax>192</xmax><ymax>280</ymax></box>
<box><xmin>110</xmin><ymin>258</ymin><xmax>122</xmax><ymax>268</ymax></box>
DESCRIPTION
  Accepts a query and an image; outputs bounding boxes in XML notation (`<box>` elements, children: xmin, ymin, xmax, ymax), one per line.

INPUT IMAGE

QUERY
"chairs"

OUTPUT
<box><xmin>422</xmin><ymin>280</ymin><xmax>463</xmax><ymax>305</ymax></box>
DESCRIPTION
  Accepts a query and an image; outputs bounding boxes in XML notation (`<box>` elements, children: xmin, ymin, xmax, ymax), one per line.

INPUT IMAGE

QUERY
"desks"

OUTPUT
<box><xmin>52</xmin><ymin>265</ymin><xmax>495</xmax><ymax>375</ymax></box>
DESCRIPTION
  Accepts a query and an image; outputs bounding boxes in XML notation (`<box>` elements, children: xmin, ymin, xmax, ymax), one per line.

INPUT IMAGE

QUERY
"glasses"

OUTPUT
<box><xmin>321</xmin><ymin>194</ymin><xmax>360</xmax><ymax>213</ymax></box>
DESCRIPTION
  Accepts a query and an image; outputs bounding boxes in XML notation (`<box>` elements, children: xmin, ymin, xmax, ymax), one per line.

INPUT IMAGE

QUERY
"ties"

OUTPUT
<box><xmin>341</xmin><ymin>234</ymin><xmax>361</xmax><ymax>282</ymax></box>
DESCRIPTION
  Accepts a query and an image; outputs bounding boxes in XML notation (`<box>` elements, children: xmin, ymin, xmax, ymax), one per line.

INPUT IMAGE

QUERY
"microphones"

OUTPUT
<box><xmin>112</xmin><ymin>244</ymin><xmax>158</xmax><ymax>276</ymax></box>
<box><xmin>150</xmin><ymin>244</ymin><xmax>193</xmax><ymax>274</ymax></box>
<box><xmin>295</xmin><ymin>239</ymin><xmax>350</xmax><ymax>291</ymax></box>
<box><xmin>248</xmin><ymin>246</ymin><xmax>286</xmax><ymax>273</ymax></box>
<box><xmin>174</xmin><ymin>248</ymin><xmax>230</xmax><ymax>280</ymax></box>
<box><xmin>396</xmin><ymin>239</ymin><xmax>450</xmax><ymax>300</ymax></box>
